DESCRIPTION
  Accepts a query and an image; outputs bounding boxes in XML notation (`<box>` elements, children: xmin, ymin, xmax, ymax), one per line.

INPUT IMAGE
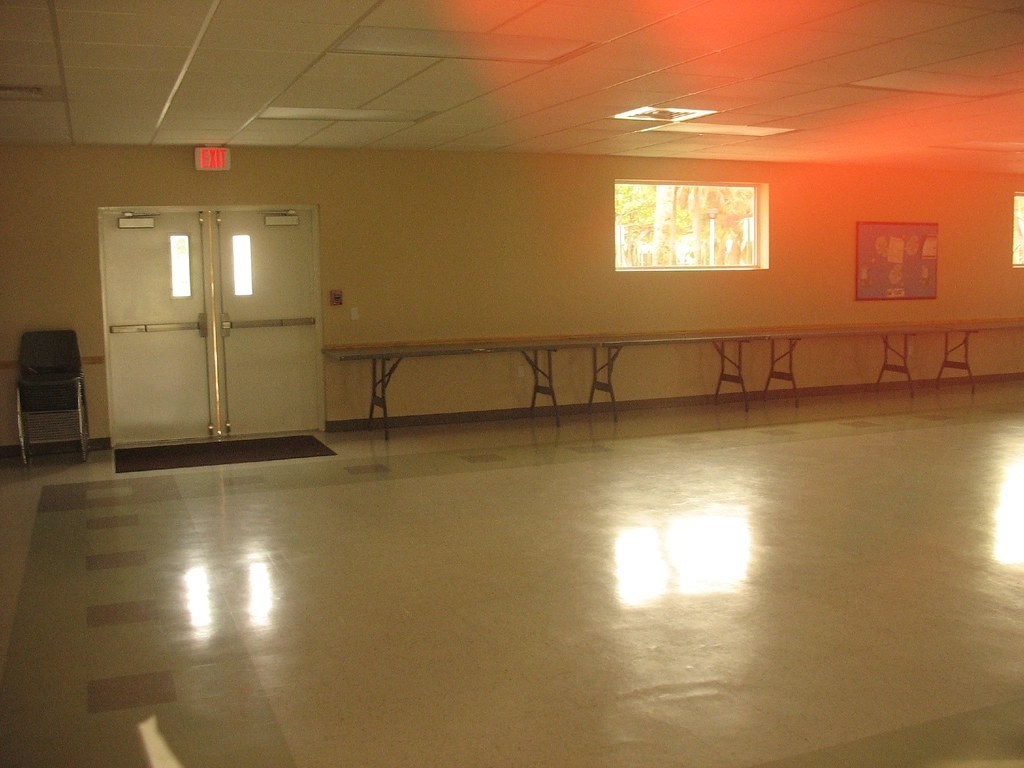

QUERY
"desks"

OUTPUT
<box><xmin>321</xmin><ymin>320</ymin><xmax>1024</xmax><ymax>442</ymax></box>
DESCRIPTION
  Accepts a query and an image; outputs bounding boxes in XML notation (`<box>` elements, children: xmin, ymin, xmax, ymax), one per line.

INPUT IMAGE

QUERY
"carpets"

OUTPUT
<box><xmin>114</xmin><ymin>435</ymin><xmax>338</xmax><ymax>473</ymax></box>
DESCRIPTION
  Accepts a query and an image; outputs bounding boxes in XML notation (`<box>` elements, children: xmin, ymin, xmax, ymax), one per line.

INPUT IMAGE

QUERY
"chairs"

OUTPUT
<box><xmin>14</xmin><ymin>330</ymin><xmax>89</xmax><ymax>465</ymax></box>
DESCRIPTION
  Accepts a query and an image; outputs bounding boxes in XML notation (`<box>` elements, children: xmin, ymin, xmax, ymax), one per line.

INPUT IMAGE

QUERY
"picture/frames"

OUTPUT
<box><xmin>854</xmin><ymin>220</ymin><xmax>939</xmax><ymax>302</ymax></box>
